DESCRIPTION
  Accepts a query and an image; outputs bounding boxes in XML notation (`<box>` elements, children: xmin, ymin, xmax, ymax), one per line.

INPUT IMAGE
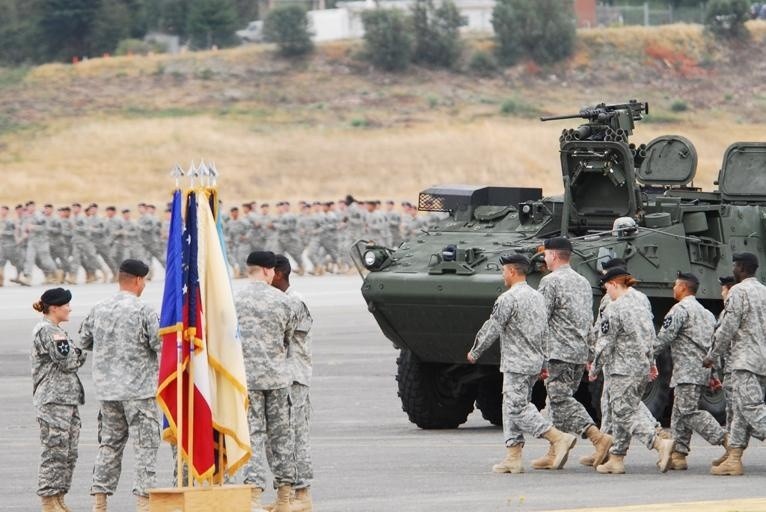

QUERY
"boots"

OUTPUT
<box><xmin>136</xmin><ymin>495</ymin><xmax>149</xmax><ymax>512</ymax></box>
<box><xmin>94</xmin><ymin>493</ymin><xmax>109</xmax><ymax>512</ymax></box>
<box><xmin>0</xmin><ymin>265</ymin><xmax>119</xmax><ymax>290</ymax></box>
<box><xmin>267</xmin><ymin>483</ymin><xmax>313</xmax><ymax>512</ymax></box>
<box><xmin>530</xmin><ymin>424</ymin><xmax>578</xmax><ymax>470</ymax></box>
<box><xmin>249</xmin><ymin>486</ymin><xmax>267</xmax><ymax>512</ymax></box>
<box><xmin>655</xmin><ymin>425</ymin><xmax>688</xmax><ymax>472</ymax></box>
<box><xmin>291</xmin><ymin>264</ymin><xmax>367</xmax><ymax>276</ymax></box>
<box><xmin>39</xmin><ymin>494</ymin><xmax>71</xmax><ymax>512</ymax></box>
<box><xmin>710</xmin><ymin>430</ymin><xmax>745</xmax><ymax>475</ymax></box>
<box><xmin>492</xmin><ymin>442</ymin><xmax>528</xmax><ymax>474</ymax></box>
<box><xmin>579</xmin><ymin>424</ymin><xmax>627</xmax><ymax>474</ymax></box>
<box><xmin>232</xmin><ymin>263</ymin><xmax>240</xmax><ymax>277</ymax></box>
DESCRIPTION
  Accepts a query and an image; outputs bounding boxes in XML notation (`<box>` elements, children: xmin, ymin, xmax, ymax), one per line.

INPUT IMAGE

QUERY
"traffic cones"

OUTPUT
<box><xmin>70</xmin><ymin>41</ymin><xmax>219</xmax><ymax>66</ymax></box>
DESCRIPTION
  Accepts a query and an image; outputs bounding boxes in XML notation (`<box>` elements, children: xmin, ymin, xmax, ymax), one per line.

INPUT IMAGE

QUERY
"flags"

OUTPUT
<box><xmin>155</xmin><ymin>185</ymin><xmax>252</xmax><ymax>488</ymax></box>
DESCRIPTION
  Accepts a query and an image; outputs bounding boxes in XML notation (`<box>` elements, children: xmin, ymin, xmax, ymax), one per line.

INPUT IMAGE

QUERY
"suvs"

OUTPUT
<box><xmin>233</xmin><ymin>19</ymin><xmax>266</xmax><ymax>44</ymax></box>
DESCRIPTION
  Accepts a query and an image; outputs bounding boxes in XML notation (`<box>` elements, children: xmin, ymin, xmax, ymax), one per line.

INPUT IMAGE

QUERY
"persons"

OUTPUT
<box><xmin>79</xmin><ymin>258</ymin><xmax>162</xmax><ymax>511</ymax></box>
<box><xmin>30</xmin><ymin>287</ymin><xmax>85</xmax><ymax>511</ymax></box>
<box><xmin>232</xmin><ymin>250</ymin><xmax>298</xmax><ymax>512</ymax></box>
<box><xmin>270</xmin><ymin>255</ymin><xmax>312</xmax><ymax>512</ymax></box>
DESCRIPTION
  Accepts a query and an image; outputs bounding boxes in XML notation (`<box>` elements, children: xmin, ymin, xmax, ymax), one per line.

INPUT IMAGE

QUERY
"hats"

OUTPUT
<box><xmin>601</xmin><ymin>257</ymin><xmax>625</xmax><ymax>270</ymax></box>
<box><xmin>718</xmin><ymin>275</ymin><xmax>735</xmax><ymax>284</ymax></box>
<box><xmin>118</xmin><ymin>259</ymin><xmax>149</xmax><ymax>277</ymax></box>
<box><xmin>542</xmin><ymin>237</ymin><xmax>573</xmax><ymax>251</ymax></box>
<box><xmin>218</xmin><ymin>195</ymin><xmax>420</xmax><ymax>214</ymax></box>
<box><xmin>247</xmin><ymin>251</ymin><xmax>277</xmax><ymax>268</ymax></box>
<box><xmin>41</xmin><ymin>287</ymin><xmax>72</xmax><ymax>306</ymax></box>
<box><xmin>677</xmin><ymin>270</ymin><xmax>695</xmax><ymax>281</ymax></box>
<box><xmin>0</xmin><ymin>200</ymin><xmax>175</xmax><ymax>216</ymax></box>
<box><xmin>732</xmin><ymin>252</ymin><xmax>758</xmax><ymax>262</ymax></box>
<box><xmin>599</xmin><ymin>268</ymin><xmax>631</xmax><ymax>286</ymax></box>
<box><xmin>499</xmin><ymin>254</ymin><xmax>529</xmax><ymax>265</ymax></box>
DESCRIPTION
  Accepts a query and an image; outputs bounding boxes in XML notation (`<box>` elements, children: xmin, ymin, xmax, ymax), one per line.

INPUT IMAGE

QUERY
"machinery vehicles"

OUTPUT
<box><xmin>348</xmin><ymin>94</ymin><xmax>766</xmax><ymax>436</ymax></box>
